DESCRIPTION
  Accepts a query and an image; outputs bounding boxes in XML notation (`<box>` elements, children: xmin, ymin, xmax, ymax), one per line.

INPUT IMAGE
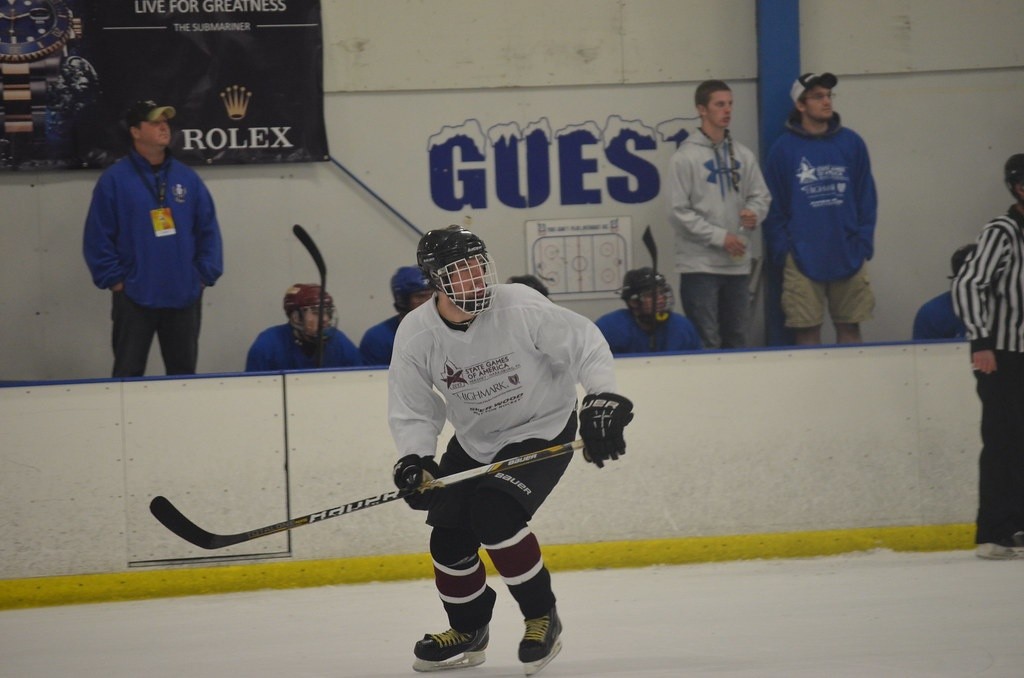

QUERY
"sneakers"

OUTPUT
<box><xmin>412</xmin><ymin>622</ymin><xmax>489</xmax><ymax>672</ymax></box>
<box><xmin>517</xmin><ymin>605</ymin><xmax>564</xmax><ymax>677</ymax></box>
<box><xmin>975</xmin><ymin>531</ymin><xmax>1024</xmax><ymax>560</ymax></box>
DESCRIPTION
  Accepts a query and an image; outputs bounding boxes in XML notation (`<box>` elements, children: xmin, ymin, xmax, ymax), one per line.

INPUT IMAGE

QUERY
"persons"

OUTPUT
<box><xmin>245</xmin><ymin>283</ymin><xmax>364</xmax><ymax>372</ymax></box>
<box><xmin>763</xmin><ymin>71</ymin><xmax>879</xmax><ymax>348</ymax></box>
<box><xmin>952</xmin><ymin>153</ymin><xmax>1024</xmax><ymax>560</ymax></box>
<box><xmin>83</xmin><ymin>100</ymin><xmax>223</xmax><ymax>378</ymax></box>
<box><xmin>671</xmin><ymin>78</ymin><xmax>771</xmax><ymax>351</ymax></box>
<box><xmin>359</xmin><ymin>267</ymin><xmax>554</xmax><ymax>371</ymax></box>
<box><xmin>387</xmin><ymin>227</ymin><xmax>633</xmax><ymax>676</ymax></box>
<box><xmin>595</xmin><ymin>266</ymin><xmax>704</xmax><ymax>355</ymax></box>
<box><xmin>913</xmin><ymin>244</ymin><xmax>978</xmax><ymax>341</ymax></box>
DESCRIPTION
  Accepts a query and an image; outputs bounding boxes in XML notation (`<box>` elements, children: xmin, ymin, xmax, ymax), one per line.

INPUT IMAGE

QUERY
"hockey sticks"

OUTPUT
<box><xmin>641</xmin><ymin>224</ymin><xmax>658</xmax><ymax>351</ymax></box>
<box><xmin>149</xmin><ymin>437</ymin><xmax>589</xmax><ymax>550</ymax></box>
<box><xmin>291</xmin><ymin>222</ymin><xmax>329</xmax><ymax>366</ymax></box>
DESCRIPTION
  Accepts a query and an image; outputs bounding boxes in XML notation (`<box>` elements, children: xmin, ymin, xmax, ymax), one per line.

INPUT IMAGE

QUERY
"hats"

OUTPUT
<box><xmin>790</xmin><ymin>72</ymin><xmax>839</xmax><ymax>102</ymax></box>
<box><xmin>125</xmin><ymin>99</ymin><xmax>177</xmax><ymax>127</ymax></box>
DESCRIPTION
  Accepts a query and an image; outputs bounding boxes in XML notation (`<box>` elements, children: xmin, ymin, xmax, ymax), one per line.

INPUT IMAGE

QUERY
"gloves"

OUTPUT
<box><xmin>392</xmin><ymin>454</ymin><xmax>447</xmax><ymax>511</ymax></box>
<box><xmin>578</xmin><ymin>392</ymin><xmax>635</xmax><ymax>469</ymax></box>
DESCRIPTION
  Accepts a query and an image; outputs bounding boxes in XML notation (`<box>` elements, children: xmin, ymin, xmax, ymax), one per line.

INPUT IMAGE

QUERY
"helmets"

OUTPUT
<box><xmin>507</xmin><ymin>274</ymin><xmax>548</xmax><ymax>299</ymax></box>
<box><xmin>390</xmin><ymin>264</ymin><xmax>435</xmax><ymax>316</ymax></box>
<box><xmin>416</xmin><ymin>224</ymin><xmax>499</xmax><ymax>316</ymax></box>
<box><xmin>947</xmin><ymin>242</ymin><xmax>979</xmax><ymax>279</ymax></box>
<box><xmin>282</xmin><ymin>283</ymin><xmax>339</xmax><ymax>347</ymax></box>
<box><xmin>1003</xmin><ymin>153</ymin><xmax>1023</xmax><ymax>205</ymax></box>
<box><xmin>619</xmin><ymin>266</ymin><xmax>675</xmax><ymax>330</ymax></box>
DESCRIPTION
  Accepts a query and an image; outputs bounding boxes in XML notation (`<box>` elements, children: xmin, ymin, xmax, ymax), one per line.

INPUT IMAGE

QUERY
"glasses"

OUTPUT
<box><xmin>804</xmin><ymin>91</ymin><xmax>836</xmax><ymax>102</ymax></box>
